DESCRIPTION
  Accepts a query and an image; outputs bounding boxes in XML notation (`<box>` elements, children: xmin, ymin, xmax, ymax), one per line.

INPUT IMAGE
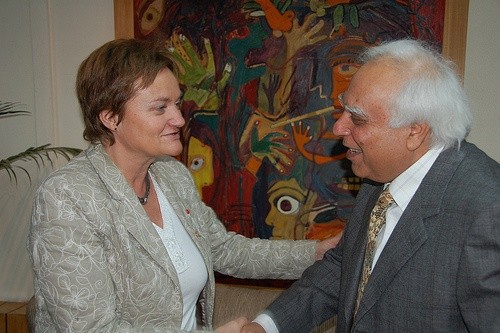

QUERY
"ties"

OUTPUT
<box><xmin>351</xmin><ymin>184</ymin><xmax>395</xmax><ymax>323</ymax></box>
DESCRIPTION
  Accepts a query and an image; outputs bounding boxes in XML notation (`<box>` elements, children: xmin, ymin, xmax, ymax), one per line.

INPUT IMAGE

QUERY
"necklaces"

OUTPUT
<box><xmin>137</xmin><ymin>174</ymin><xmax>151</xmax><ymax>204</ymax></box>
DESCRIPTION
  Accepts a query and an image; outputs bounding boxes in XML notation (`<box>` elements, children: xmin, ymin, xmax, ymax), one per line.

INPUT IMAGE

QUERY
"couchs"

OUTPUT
<box><xmin>26</xmin><ymin>283</ymin><xmax>339</xmax><ymax>333</ymax></box>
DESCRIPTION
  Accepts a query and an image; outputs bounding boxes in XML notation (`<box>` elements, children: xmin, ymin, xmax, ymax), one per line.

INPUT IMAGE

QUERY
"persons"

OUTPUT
<box><xmin>241</xmin><ymin>35</ymin><xmax>500</xmax><ymax>333</ymax></box>
<box><xmin>29</xmin><ymin>39</ymin><xmax>344</xmax><ymax>333</ymax></box>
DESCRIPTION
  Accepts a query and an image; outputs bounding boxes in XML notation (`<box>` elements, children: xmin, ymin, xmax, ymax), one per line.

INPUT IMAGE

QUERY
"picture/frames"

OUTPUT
<box><xmin>113</xmin><ymin>0</ymin><xmax>470</xmax><ymax>290</ymax></box>
